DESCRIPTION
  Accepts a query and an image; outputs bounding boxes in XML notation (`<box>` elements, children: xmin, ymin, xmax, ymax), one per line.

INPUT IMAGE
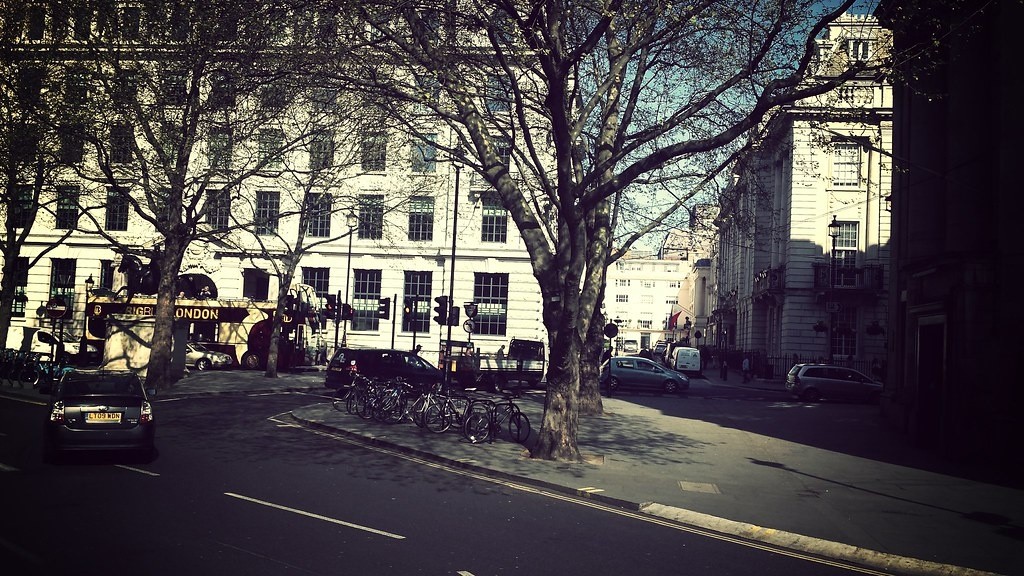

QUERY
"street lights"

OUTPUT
<box><xmin>341</xmin><ymin>207</ymin><xmax>357</xmax><ymax>349</ymax></box>
<box><xmin>443</xmin><ymin>138</ymin><xmax>467</xmax><ymax>384</ymax></box>
<box><xmin>79</xmin><ymin>274</ymin><xmax>94</xmax><ymax>354</ymax></box>
<box><xmin>829</xmin><ymin>215</ymin><xmax>841</xmax><ymax>362</ymax></box>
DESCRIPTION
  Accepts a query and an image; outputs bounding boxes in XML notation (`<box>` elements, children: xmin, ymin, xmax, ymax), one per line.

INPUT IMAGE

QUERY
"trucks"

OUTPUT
<box><xmin>451</xmin><ymin>338</ymin><xmax>545</xmax><ymax>393</ymax></box>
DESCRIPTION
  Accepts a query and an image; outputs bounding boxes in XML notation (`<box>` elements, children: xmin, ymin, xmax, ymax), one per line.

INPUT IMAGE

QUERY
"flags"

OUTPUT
<box><xmin>667</xmin><ymin>305</ymin><xmax>682</xmax><ymax>331</ymax></box>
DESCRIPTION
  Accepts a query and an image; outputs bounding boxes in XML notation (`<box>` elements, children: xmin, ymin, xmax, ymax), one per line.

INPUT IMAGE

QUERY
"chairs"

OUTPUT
<box><xmin>114</xmin><ymin>379</ymin><xmax>131</xmax><ymax>392</ymax></box>
<box><xmin>66</xmin><ymin>381</ymin><xmax>85</xmax><ymax>394</ymax></box>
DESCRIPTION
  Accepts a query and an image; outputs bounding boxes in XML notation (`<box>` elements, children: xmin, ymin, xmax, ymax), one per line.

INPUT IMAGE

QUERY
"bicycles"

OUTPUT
<box><xmin>0</xmin><ymin>347</ymin><xmax>69</xmax><ymax>388</ymax></box>
<box><xmin>331</xmin><ymin>369</ymin><xmax>531</xmax><ymax>444</ymax></box>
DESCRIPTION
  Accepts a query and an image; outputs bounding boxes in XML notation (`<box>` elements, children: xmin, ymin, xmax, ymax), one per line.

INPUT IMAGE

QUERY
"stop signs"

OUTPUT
<box><xmin>46</xmin><ymin>298</ymin><xmax>67</xmax><ymax>319</ymax></box>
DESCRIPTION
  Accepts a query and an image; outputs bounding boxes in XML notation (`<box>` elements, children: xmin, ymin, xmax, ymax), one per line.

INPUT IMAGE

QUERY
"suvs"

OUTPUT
<box><xmin>785</xmin><ymin>362</ymin><xmax>883</xmax><ymax>402</ymax></box>
<box><xmin>5</xmin><ymin>326</ymin><xmax>99</xmax><ymax>364</ymax></box>
<box><xmin>325</xmin><ymin>347</ymin><xmax>457</xmax><ymax>398</ymax></box>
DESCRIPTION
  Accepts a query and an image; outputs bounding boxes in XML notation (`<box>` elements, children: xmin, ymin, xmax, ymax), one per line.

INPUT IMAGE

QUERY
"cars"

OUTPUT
<box><xmin>599</xmin><ymin>355</ymin><xmax>689</xmax><ymax>393</ymax></box>
<box><xmin>40</xmin><ymin>368</ymin><xmax>155</xmax><ymax>457</ymax></box>
<box><xmin>184</xmin><ymin>341</ymin><xmax>234</xmax><ymax>370</ymax></box>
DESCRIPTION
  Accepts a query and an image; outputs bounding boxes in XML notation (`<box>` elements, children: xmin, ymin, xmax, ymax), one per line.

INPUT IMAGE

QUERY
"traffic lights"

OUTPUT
<box><xmin>343</xmin><ymin>304</ymin><xmax>352</xmax><ymax>320</ymax></box>
<box><xmin>433</xmin><ymin>296</ymin><xmax>447</xmax><ymax>324</ymax></box>
<box><xmin>404</xmin><ymin>298</ymin><xmax>413</xmax><ymax>322</ymax></box>
<box><xmin>324</xmin><ymin>294</ymin><xmax>336</xmax><ymax>320</ymax></box>
<box><xmin>378</xmin><ymin>298</ymin><xmax>390</xmax><ymax>320</ymax></box>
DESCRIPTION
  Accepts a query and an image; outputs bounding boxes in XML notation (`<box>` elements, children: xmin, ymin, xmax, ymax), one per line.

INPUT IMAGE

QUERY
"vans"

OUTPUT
<box><xmin>652</xmin><ymin>341</ymin><xmax>701</xmax><ymax>372</ymax></box>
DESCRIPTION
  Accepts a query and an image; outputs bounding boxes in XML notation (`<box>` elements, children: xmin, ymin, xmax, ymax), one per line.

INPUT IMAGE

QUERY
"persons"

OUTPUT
<box><xmin>601</xmin><ymin>346</ymin><xmax>614</xmax><ymax>364</ymax></box>
<box><xmin>319</xmin><ymin>335</ymin><xmax>330</xmax><ymax>365</ymax></box>
<box><xmin>496</xmin><ymin>345</ymin><xmax>505</xmax><ymax>368</ymax></box>
<box><xmin>791</xmin><ymin>353</ymin><xmax>799</xmax><ymax>369</ymax></box>
<box><xmin>701</xmin><ymin>344</ymin><xmax>721</xmax><ymax>368</ymax></box>
<box><xmin>741</xmin><ymin>355</ymin><xmax>750</xmax><ymax>383</ymax></box>
<box><xmin>846</xmin><ymin>356</ymin><xmax>855</xmax><ymax>369</ymax></box>
<box><xmin>639</xmin><ymin>346</ymin><xmax>649</xmax><ymax>355</ymax></box>
<box><xmin>408</xmin><ymin>344</ymin><xmax>423</xmax><ymax>363</ymax></box>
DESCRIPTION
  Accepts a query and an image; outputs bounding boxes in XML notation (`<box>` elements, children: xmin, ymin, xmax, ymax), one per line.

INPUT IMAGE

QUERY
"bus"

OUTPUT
<box><xmin>84</xmin><ymin>295</ymin><xmax>318</xmax><ymax>374</ymax></box>
